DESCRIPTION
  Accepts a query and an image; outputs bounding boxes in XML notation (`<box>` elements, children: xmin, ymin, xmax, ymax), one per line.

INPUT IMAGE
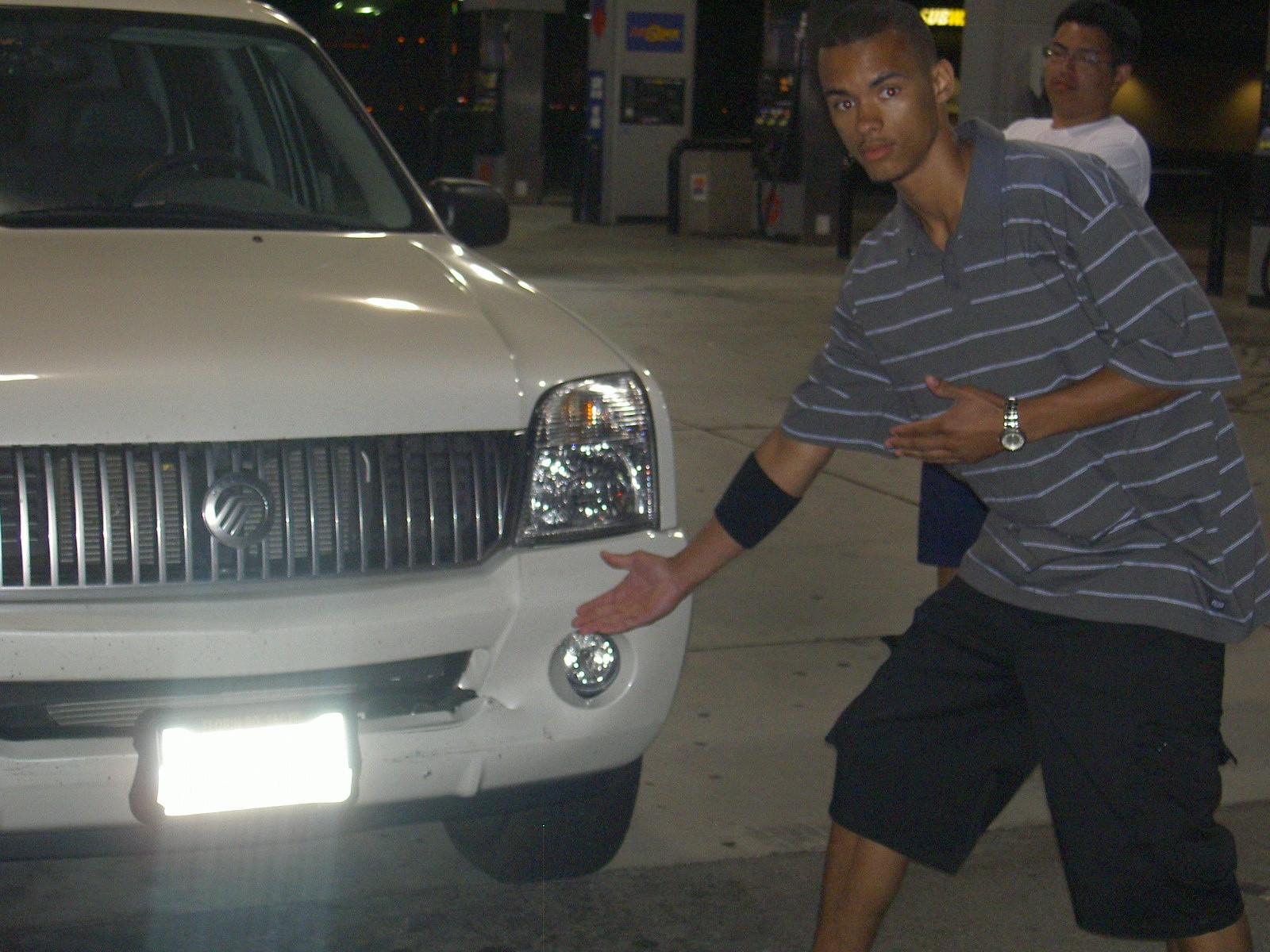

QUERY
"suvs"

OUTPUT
<box><xmin>1</xmin><ymin>0</ymin><xmax>689</xmax><ymax>884</ymax></box>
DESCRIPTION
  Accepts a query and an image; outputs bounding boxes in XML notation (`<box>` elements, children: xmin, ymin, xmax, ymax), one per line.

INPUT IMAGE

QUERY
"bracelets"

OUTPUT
<box><xmin>711</xmin><ymin>453</ymin><xmax>804</xmax><ymax>554</ymax></box>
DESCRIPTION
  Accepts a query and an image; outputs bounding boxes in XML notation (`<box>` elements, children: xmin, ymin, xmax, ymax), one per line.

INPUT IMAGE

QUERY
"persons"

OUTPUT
<box><xmin>566</xmin><ymin>0</ymin><xmax>1269</xmax><ymax>951</ymax></box>
<box><xmin>914</xmin><ymin>2</ymin><xmax>1157</xmax><ymax>585</ymax></box>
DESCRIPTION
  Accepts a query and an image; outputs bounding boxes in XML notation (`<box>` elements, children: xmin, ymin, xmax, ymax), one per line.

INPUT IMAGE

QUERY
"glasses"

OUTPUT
<box><xmin>1042</xmin><ymin>44</ymin><xmax>1121</xmax><ymax>66</ymax></box>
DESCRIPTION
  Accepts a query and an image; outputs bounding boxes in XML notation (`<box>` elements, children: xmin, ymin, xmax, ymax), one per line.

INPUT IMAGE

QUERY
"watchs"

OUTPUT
<box><xmin>1001</xmin><ymin>396</ymin><xmax>1026</xmax><ymax>453</ymax></box>
